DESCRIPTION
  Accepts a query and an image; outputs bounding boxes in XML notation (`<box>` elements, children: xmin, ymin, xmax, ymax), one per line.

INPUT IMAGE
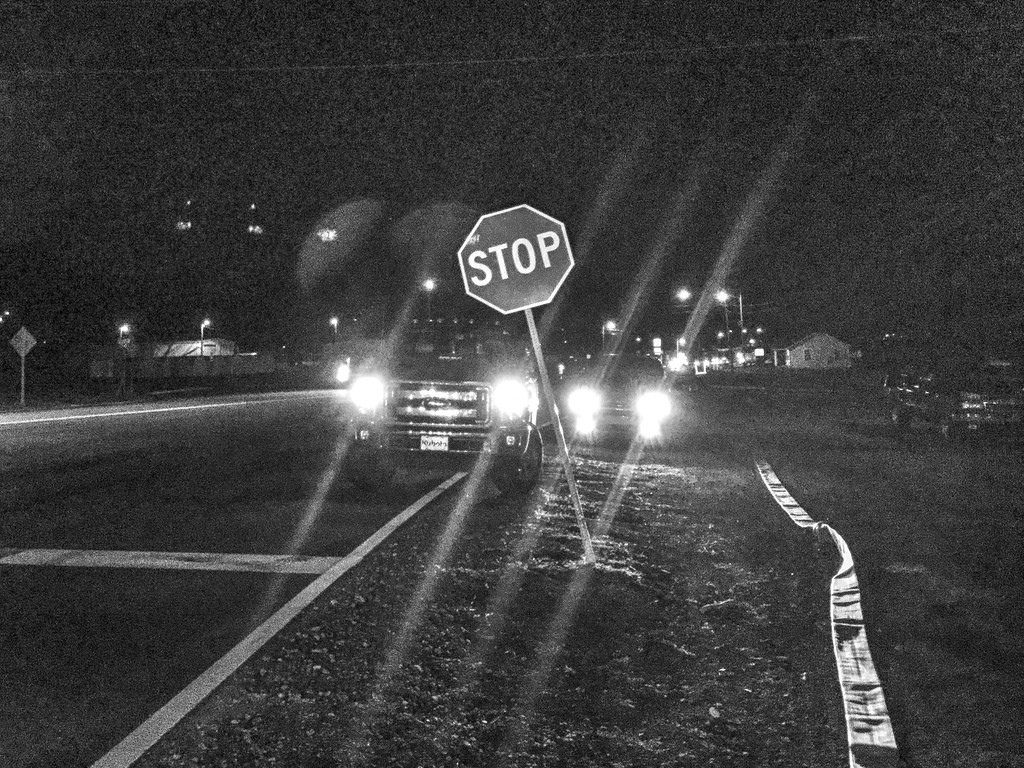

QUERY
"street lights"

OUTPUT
<box><xmin>713</xmin><ymin>290</ymin><xmax>735</xmax><ymax>374</ymax></box>
<box><xmin>601</xmin><ymin>321</ymin><xmax>617</xmax><ymax>355</ymax></box>
<box><xmin>199</xmin><ymin>318</ymin><xmax>210</xmax><ymax>356</ymax></box>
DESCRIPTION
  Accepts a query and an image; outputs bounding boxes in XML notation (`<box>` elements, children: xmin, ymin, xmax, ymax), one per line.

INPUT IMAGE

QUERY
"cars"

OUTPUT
<box><xmin>882</xmin><ymin>368</ymin><xmax>1024</xmax><ymax>443</ymax></box>
<box><xmin>566</xmin><ymin>353</ymin><xmax>675</xmax><ymax>441</ymax></box>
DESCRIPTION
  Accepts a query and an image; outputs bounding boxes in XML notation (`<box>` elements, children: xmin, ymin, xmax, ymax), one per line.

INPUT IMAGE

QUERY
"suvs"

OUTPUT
<box><xmin>342</xmin><ymin>316</ymin><xmax>575</xmax><ymax>496</ymax></box>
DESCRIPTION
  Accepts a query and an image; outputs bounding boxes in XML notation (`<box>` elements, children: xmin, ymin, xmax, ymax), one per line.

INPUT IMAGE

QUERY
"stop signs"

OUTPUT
<box><xmin>455</xmin><ymin>204</ymin><xmax>577</xmax><ymax>318</ymax></box>
